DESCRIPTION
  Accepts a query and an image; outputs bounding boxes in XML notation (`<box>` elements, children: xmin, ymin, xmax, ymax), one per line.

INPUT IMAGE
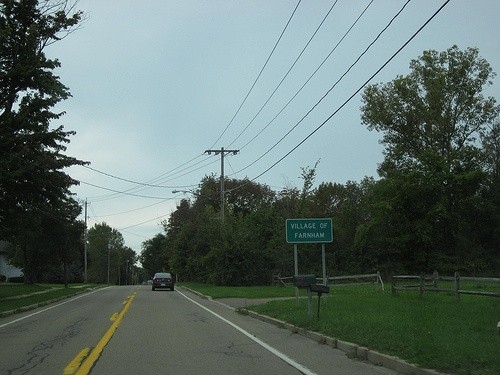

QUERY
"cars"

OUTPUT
<box><xmin>152</xmin><ymin>272</ymin><xmax>175</xmax><ymax>291</ymax></box>
